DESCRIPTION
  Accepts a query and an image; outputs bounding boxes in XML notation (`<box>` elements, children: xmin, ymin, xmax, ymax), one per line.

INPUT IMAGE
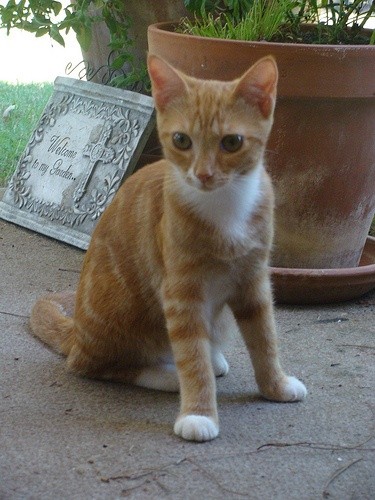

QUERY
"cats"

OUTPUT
<box><xmin>26</xmin><ymin>51</ymin><xmax>307</xmax><ymax>441</ymax></box>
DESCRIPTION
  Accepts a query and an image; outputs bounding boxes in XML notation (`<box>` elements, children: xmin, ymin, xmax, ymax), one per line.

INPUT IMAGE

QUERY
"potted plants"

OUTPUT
<box><xmin>0</xmin><ymin>0</ymin><xmax>199</xmax><ymax>99</ymax></box>
<box><xmin>146</xmin><ymin>0</ymin><xmax>375</xmax><ymax>270</ymax></box>
